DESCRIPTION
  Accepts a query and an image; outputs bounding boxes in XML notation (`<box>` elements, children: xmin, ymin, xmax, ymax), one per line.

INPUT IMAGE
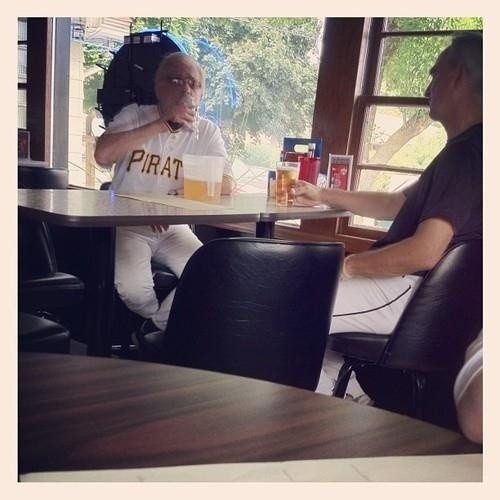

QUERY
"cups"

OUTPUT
<box><xmin>276</xmin><ymin>162</ymin><xmax>300</xmax><ymax>206</ymax></box>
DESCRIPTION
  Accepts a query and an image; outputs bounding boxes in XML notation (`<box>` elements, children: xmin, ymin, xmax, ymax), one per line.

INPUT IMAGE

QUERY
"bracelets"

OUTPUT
<box><xmin>342</xmin><ymin>254</ymin><xmax>355</xmax><ymax>279</ymax></box>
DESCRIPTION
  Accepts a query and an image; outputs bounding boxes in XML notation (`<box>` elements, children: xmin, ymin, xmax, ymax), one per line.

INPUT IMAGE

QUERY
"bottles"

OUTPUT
<box><xmin>297</xmin><ymin>142</ymin><xmax>321</xmax><ymax>189</ymax></box>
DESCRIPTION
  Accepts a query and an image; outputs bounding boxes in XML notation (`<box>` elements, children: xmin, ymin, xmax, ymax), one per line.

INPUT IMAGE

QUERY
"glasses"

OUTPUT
<box><xmin>157</xmin><ymin>76</ymin><xmax>202</xmax><ymax>92</ymax></box>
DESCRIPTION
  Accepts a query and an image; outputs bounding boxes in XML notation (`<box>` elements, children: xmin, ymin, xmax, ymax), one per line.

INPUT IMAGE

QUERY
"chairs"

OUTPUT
<box><xmin>329</xmin><ymin>239</ymin><xmax>483</xmax><ymax>421</ymax></box>
<box><xmin>17</xmin><ymin>165</ymin><xmax>86</xmax><ymax>296</ymax></box>
<box><xmin>162</xmin><ymin>236</ymin><xmax>346</xmax><ymax>391</ymax></box>
<box><xmin>99</xmin><ymin>181</ymin><xmax>178</xmax><ymax>291</ymax></box>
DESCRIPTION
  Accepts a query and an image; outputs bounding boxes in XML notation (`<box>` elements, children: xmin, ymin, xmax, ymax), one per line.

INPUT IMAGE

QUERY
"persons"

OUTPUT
<box><xmin>94</xmin><ymin>53</ymin><xmax>236</xmax><ymax>355</ymax></box>
<box><xmin>291</xmin><ymin>34</ymin><xmax>483</xmax><ymax>335</ymax></box>
<box><xmin>454</xmin><ymin>329</ymin><xmax>483</xmax><ymax>445</ymax></box>
